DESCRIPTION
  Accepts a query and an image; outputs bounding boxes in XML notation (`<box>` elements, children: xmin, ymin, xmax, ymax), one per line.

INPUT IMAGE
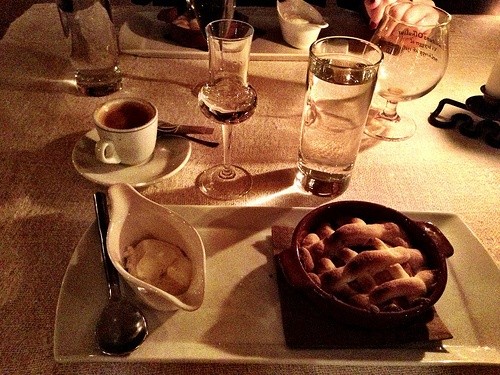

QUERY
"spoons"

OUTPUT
<box><xmin>92</xmin><ymin>193</ymin><xmax>149</xmax><ymax>358</ymax></box>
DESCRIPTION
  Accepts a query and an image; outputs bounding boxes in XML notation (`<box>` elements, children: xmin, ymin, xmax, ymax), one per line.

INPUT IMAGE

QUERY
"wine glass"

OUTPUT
<box><xmin>199</xmin><ymin>18</ymin><xmax>258</xmax><ymax>200</ymax></box>
<box><xmin>356</xmin><ymin>1</ymin><xmax>452</xmax><ymax>145</ymax></box>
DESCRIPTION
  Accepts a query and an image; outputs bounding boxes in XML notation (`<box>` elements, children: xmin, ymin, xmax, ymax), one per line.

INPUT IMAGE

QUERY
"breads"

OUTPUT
<box><xmin>299</xmin><ymin>214</ymin><xmax>440</xmax><ymax>313</ymax></box>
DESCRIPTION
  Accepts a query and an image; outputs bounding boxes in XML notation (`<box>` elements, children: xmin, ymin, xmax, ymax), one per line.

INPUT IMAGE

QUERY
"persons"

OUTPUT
<box><xmin>364</xmin><ymin>0</ymin><xmax>439</xmax><ymax>49</ymax></box>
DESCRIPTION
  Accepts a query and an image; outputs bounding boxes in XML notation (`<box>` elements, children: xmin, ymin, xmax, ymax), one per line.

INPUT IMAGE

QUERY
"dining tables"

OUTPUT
<box><xmin>0</xmin><ymin>0</ymin><xmax>500</xmax><ymax>373</ymax></box>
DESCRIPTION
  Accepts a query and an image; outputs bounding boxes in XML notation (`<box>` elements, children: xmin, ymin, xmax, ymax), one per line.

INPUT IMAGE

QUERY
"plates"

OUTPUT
<box><xmin>71</xmin><ymin>119</ymin><xmax>192</xmax><ymax>188</ymax></box>
<box><xmin>53</xmin><ymin>203</ymin><xmax>499</xmax><ymax>365</ymax></box>
<box><xmin>118</xmin><ymin>11</ymin><xmax>349</xmax><ymax>61</ymax></box>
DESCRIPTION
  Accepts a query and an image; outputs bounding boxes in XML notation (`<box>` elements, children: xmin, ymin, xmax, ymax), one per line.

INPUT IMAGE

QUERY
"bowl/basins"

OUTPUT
<box><xmin>276</xmin><ymin>0</ymin><xmax>329</xmax><ymax>50</ymax></box>
<box><xmin>156</xmin><ymin>4</ymin><xmax>249</xmax><ymax>47</ymax></box>
<box><xmin>105</xmin><ymin>182</ymin><xmax>206</xmax><ymax>312</ymax></box>
<box><xmin>277</xmin><ymin>201</ymin><xmax>454</xmax><ymax>326</ymax></box>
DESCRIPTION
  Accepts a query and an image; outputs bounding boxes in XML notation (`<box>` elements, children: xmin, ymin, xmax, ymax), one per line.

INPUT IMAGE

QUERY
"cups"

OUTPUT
<box><xmin>296</xmin><ymin>36</ymin><xmax>385</xmax><ymax>198</ymax></box>
<box><xmin>92</xmin><ymin>98</ymin><xmax>159</xmax><ymax>166</ymax></box>
<box><xmin>55</xmin><ymin>0</ymin><xmax>123</xmax><ymax>97</ymax></box>
